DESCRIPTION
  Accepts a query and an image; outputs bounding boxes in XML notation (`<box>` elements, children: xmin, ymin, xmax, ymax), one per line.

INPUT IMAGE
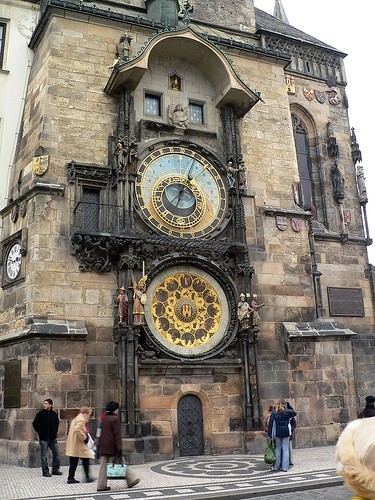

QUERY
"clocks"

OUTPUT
<box><xmin>3</xmin><ymin>239</ymin><xmax>21</xmax><ymax>281</ymax></box>
<box><xmin>131</xmin><ymin>137</ymin><xmax>236</xmax><ymax>241</ymax></box>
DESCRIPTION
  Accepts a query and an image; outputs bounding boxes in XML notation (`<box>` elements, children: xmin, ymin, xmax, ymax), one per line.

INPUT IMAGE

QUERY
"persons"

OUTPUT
<box><xmin>238</xmin><ymin>292</ymin><xmax>265</xmax><ymax>331</ymax></box>
<box><xmin>115</xmin><ymin>143</ymin><xmax>138</xmax><ymax>174</ymax></box>
<box><xmin>266</xmin><ymin>400</ymin><xmax>296</xmax><ymax>472</ymax></box>
<box><xmin>97</xmin><ymin>401</ymin><xmax>141</xmax><ymax>492</ymax></box>
<box><xmin>335</xmin><ymin>396</ymin><xmax>375</xmax><ymax>500</ymax></box>
<box><xmin>168</xmin><ymin>104</ymin><xmax>189</xmax><ymax>128</ymax></box>
<box><xmin>66</xmin><ymin>406</ymin><xmax>97</xmax><ymax>484</ymax></box>
<box><xmin>117</xmin><ymin>275</ymin><xmax>148</xmax><ymax>325</ymax></box>
<box><xmin>32</xmin><ymin>399</ymin><xmax>63</xmax><ymax>477</ymax></box>
<box><xmin>226</xmin><ymin>161</ymin><xmax>247</xmax><ymax>189</ymax></box>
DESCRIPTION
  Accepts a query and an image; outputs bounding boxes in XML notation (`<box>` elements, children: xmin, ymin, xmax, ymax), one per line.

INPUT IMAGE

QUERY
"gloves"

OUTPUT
<box><xmin>84</xmin><ymin>434</ymin><xmax>89</xmax><ymax>444</ymax></box>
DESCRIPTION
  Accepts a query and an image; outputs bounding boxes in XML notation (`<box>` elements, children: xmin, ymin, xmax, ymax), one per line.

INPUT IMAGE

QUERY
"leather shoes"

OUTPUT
<box><xmin>43</xmin><ymin>472</ymin><xmax>51</xmax><ymax>477</ymax></box>
<box><xmin>52</xmin><ymin>471</ymin><xmax>62</xmax><ymax>475</ymax></box>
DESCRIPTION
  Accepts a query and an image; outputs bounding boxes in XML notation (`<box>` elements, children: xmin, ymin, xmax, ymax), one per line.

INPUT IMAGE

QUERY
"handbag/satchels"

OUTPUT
<box><xmin>264</xmin><ymin>438</ymin><xmax>277</xmax><ymax>464</ymax></box>
<box><xmin>106</xmin><ymin>451</ymin><xmax>127</xmax><ymax>479</ymax></box>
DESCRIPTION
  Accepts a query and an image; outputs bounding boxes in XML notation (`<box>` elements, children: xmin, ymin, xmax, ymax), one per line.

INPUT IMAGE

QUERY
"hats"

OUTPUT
<box><xmin>334</xmin><ymin>416</ymin><xmax>375</xmax><ymax>500</ymax></box>
<box><xmin>365</xmin><ymin>396</ymin><xmax>375</xmax><ymax>402</ymax></box>
<box><xmin>106</xmin><ymin>401</ymin><xmax>119</xmax><ymax>411</ymax></box>
<box><xmin>80</xmin><ymin>406</ymin><xmax>92</xmax><ymax>412</ymax></box>
<box><xmin>268</xmin><ymin>406</ymin><xmax>277</xmax><ymax>411</ymax></box>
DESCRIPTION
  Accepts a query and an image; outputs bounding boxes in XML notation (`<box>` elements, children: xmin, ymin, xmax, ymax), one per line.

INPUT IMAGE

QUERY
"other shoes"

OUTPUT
<box><xmin>280</xmin><ymin>468</ymin><xmax>287</xmax><ymax>472</ymax></box>
<box><xmin>67</xmin><ymin>479</ymin><xmax>79</xmax><ymax>484</ymax></box>
<box><xmin>97</xmin><ymin>487</ymin><xmax>111</xmax><ymax>491</ymax></box>
<box><xmin>270</xmin><ymin>465</ymin><xmax>278</xmax><ymax>470</ymax></box>
<box><xmin>289</xmin><ymin>462</ymin><xmax>293</xmax><ymax>465</ymax></box>
<box><xmin>128</xmin><ymin>478</ymin><xmax>140</xmax><ymax>488</ymax></box>
<box><xmin>87</xmin><ymin>479</ymin><xmax>94</xmax><ymax>482</ymax></box>
